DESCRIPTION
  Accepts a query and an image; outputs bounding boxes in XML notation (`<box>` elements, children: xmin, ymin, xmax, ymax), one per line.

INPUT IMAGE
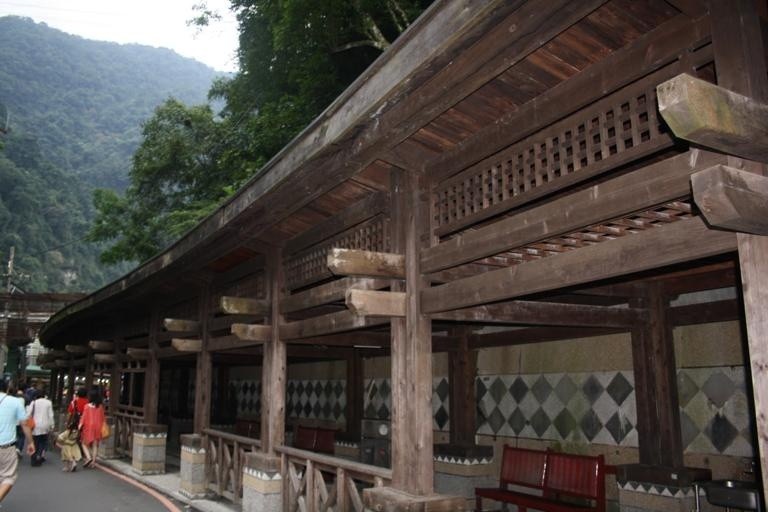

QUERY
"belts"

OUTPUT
<box><xmin>0</xmin><ymin>441</ymin><xmax>18</xmax><ymax>449</ymax></box>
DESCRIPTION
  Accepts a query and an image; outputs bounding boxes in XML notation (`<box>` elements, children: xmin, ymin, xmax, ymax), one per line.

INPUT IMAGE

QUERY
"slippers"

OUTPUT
<box><xmin>82</xmin><ymin>456</ymin><xmax>93</xmax><ymax>467</ymax></box>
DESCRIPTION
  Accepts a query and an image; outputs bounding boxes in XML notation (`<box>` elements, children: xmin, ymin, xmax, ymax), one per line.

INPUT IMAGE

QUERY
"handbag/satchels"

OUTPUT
<box><xmin>26</xmin><ymin>417</ymin><xmax>35</xmax><ymax>430</ymax></box>
<box><xmin>101</xmin><ymin>421</ymin><xmax>110</xmax><ymax>439</ymax></box>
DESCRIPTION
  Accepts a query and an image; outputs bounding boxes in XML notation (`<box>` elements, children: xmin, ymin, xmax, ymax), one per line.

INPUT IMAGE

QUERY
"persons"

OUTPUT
<box><xmin>0</xmin><ymin>380</ymin><xmax>110</xmax><ymax>508</ymax></box>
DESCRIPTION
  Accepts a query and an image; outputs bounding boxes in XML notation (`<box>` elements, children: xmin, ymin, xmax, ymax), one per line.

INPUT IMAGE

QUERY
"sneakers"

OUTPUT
<box><xmin>61</xmin><ymin>463</ymin><xmax>78</xmax><ymax>473</ymax></box>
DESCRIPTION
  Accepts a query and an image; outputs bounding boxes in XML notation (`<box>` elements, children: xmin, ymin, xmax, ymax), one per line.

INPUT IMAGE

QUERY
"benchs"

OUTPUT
<box><xmin>290</xmin><ymin>425</ymin><xmax>338</xmax><ymax>482</ymax></box>
<box><xmin>473</xmin><ymin>443</ymin><xmax>606</xmax><ymax>512</ymax></box>
<box><xmin>236</xmin><ymin>420</ymin><xmax>260</xmax><ymax>438</ymax></box>
